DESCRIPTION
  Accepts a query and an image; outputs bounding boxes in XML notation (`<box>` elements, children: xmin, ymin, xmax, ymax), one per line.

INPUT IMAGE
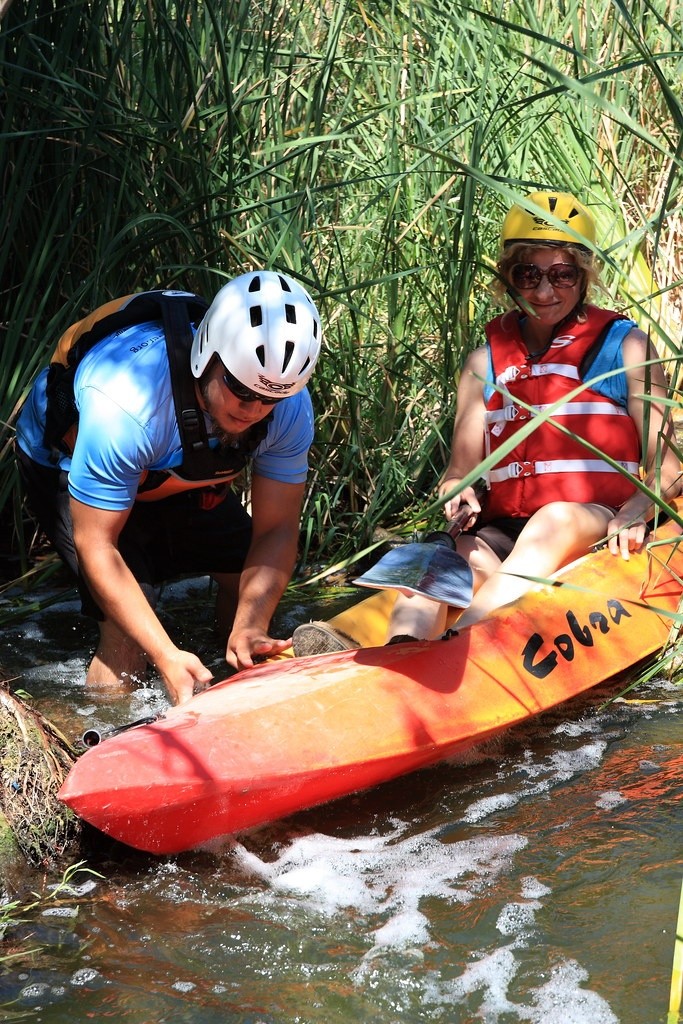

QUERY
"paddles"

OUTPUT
<box><xmin>350</xmin><ymin>477</ymin><xmax>492</xmax><ymax>609</ymax></box>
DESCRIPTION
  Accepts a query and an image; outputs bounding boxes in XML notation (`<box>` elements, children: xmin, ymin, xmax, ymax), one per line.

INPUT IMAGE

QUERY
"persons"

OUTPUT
<box><xmin>292</xmin><ymin>188</ymin><xmax>683</xmax><ymax>658</ymax></box>
<box><xmin>18</xmin><ymin>269</ymin><xmax>324</xmax><ymax>706</ymax></box>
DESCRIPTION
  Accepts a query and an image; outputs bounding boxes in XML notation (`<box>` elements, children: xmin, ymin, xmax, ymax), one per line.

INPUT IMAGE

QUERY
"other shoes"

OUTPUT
<box><xmin>292</xmin><ymin>621</ymin><xmax>360</xmax><ymax>658</ymax></box>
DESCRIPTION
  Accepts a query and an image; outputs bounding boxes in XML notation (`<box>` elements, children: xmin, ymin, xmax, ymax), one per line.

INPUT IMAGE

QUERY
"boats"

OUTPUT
<box><xmin>56</xmin><ymin>472</ymin><xmax>683</xmax><ymax>856</ymax></box>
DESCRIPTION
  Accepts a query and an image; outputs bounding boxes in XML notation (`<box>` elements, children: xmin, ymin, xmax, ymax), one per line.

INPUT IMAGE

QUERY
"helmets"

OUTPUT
<box><xmin>190</xmin><ymin>272</ymin><xmax>322</xmax><ymax>399</ymax></box>
<box><xmin>501</xmin><ymin>191</ymin><xmax>595</xmax><ymax>257</ymax></box>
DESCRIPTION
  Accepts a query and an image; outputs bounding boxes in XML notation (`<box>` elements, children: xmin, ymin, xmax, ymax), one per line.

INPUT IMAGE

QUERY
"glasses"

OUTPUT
<box><xmin>511</xmin><ymin>263</ymin><xmax>586</xmax><ymax>290</ymax></box>
<box><xmin>217</xmin><ymin>358</ymin><xmax>285</xmax><ymax>406</ymax></box>
